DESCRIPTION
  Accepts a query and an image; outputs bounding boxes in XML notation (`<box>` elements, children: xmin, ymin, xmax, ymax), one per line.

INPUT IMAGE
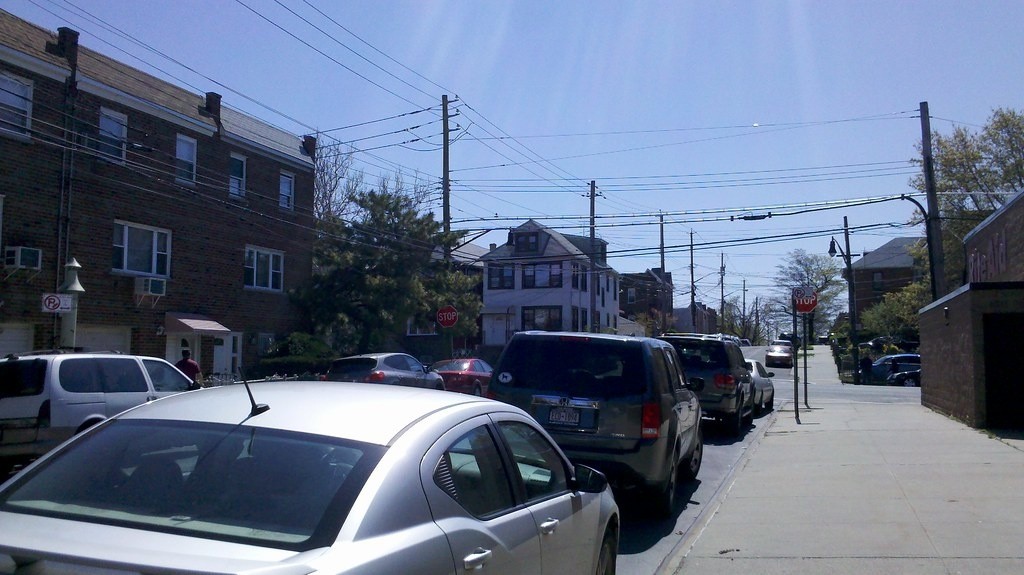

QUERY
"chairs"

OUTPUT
<box><xmin>677</xmin><ymin>350</ymin><xmax>719</xmax><ymax>369</ymax></box>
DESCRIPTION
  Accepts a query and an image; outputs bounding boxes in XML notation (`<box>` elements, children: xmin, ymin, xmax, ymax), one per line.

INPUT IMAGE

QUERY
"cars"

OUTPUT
<box><xmin>771</xmin><ymin>340</ymin><xmax>792</xmax><ymax>346</ymax></box>
<box><xmin>722</xmin><ymin>335</ymin><xmax>752</xmax><ymax>346</ymax></box>
<box><xmin>428</xmin><ymin>358</ymin><xmax>494</xmax><ymax>398</ymax></box>
<box><xmin>826</xmin><ymin>333</ymin><xmax>835</xmax><ymax>343</ymax></box>
<box><xmin>745</xmin><ymin>359</ymin><xmax>775</xmax><ymax>416</ymax></box>
<box><xmin>859</xmin><ymin>353</ymin><xmax>921</xmax><ymax>384</ymax></box>
<box><xmin>0</xmin><ymin>380</ymin><xmax>620</xmax><ymax>575</ymax></box>
<box><xmin>765</xmin><ymin>345</ymin><xmax>793</xmax><ymax>368</ymax></box>
<box><xmin>778</xmin><ymin>332</ymin><xmax>801</xmax><ymax>348</ymax></box>
<box><xmin>859</xmin><ymin>336</ymin><xmax>919</xmax><ymax>351</ymax></box>
<box><xmin>886</xmin><ymin>369</ymin><xmax>921</xmax><ymax>387</ymax></box>
<box><xmin>818</xmin><ymin>336</ymin><xmax>827</xmax><ymax>343</ymax></box>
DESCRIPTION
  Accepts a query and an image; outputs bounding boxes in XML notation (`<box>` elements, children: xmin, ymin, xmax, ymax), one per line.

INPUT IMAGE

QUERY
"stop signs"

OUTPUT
<box><xmin>436</xmin><ymin>305</ymin><xmax>459</xmax><ymax>330</ymax></box>
<box><xmin>795</xmin><ymin>290</ymin><xmax>818</xmax><ymax>313</ymax></box>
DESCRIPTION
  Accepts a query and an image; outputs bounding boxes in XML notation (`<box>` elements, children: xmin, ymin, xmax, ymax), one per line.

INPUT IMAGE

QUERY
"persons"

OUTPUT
<box><xmin>860</xmin><ymin>352</ymin><xmax>873</xmax><ymax>384</ymax></box>
<box><xmin>174</xmin><ymin>350</ymin><xmax>205</xmax><ymax>387</ymax></box>
<box><xmin>887</xmin><ymin>357</ymin><xmax>899</xmax><ymax>374</ymax></box>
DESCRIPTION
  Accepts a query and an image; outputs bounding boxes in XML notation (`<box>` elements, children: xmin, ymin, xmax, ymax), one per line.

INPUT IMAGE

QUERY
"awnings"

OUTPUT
<box><xmin>165</xmin><ymin>312</ymin><xmax>231</xmax><ymax>337</ymax></box>
<box><xmin>479</xmin><ymin>305</ymin><xmax>515</xmax><ymax>314</ymax></box>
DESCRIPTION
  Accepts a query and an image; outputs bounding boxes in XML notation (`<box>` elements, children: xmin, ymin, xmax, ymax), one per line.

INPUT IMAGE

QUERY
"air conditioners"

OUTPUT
<box><xmin>135</xmin><ymin>277</ymin><xmax>166</xmax><ymax>296</ymax></box>
<box><xmin>5</xmin><ymin>246</ymin><xmax>42</xmax><ymax>270</ymax></box>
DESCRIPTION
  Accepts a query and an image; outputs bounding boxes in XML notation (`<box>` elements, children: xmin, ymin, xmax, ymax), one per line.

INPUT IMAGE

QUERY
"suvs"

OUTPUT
<box><xmin>486</xmin><ymin>331</ymin><xmax>703</xmax><ymax>515</ymax></box>
<box><xmin>325</xmin><ymin>352</ymin><xmax>446</xmax><ymax>391</ymax></box>
<box><xmin>654</xmin><ymin>333</ymin><xmax>756</xmax><ymax>433</ymax></box>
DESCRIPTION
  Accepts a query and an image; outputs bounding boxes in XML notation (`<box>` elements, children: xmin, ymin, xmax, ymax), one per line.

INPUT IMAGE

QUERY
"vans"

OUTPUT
<box><xmin>0</xmin><ymin>346</ymin><xmax>205</xmax><ymax>478</ymax></box>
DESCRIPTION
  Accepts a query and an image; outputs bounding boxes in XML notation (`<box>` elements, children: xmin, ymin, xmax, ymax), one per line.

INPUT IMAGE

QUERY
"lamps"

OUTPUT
<box><xmin>157</xmin><ymin>325</ymin><xmax>166</xmax><ymax>335</ymax></box>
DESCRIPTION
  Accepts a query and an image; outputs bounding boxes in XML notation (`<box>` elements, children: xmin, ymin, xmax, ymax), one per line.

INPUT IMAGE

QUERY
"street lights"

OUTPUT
<box><xmin>827</xmin><ymin>236</ymin><xmax>860</xmax><ymax>385</ymax></box>
